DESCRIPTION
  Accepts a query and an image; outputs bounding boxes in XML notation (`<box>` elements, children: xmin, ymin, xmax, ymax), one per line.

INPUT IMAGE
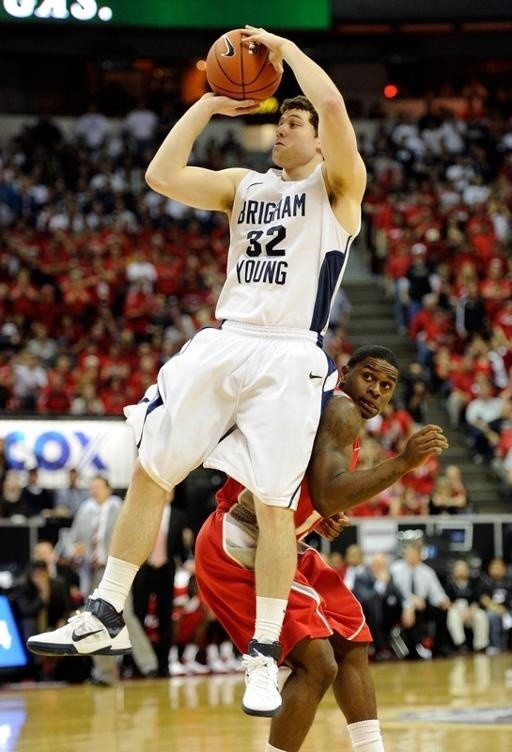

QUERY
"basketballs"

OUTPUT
<box><xmin>206</xmin><ymin>29</ymin><xmax>284</xmax><ymax>100</ymax></box>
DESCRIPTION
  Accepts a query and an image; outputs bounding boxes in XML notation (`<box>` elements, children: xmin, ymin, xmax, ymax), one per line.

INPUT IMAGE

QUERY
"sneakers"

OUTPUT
<box><xmin>366</xmin><ymin>643</ymin><xmax>502</xmax><ymax>661</ymax></box>
<box><xmin>168</xmin><ymin>654</ymin><xmax>238</xmax><ymax>677</ymax></box>
<box><xmin>26</xmin><ymin>597</ymin><xmax>132</xmax><ymax>657</ymax></box>
<box><xmin>238</xmin><ymin>636</ymin><xmax>284</xmax><ymax>719</ymax></box>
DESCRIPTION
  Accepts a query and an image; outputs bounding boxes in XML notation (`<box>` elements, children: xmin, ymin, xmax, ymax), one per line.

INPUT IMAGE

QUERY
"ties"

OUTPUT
<box><xmin>409</xmin><ymin>569</ymin><xmax>419</xmax><ymax>595</ymax></box>
<box><xmin>89</xmin><ymin>509</ymin><xmax>100</xmax><ymax>571</ymax></box>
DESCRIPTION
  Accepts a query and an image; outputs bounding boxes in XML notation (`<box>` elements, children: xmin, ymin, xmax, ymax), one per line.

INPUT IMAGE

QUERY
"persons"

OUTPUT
<box><xmin>1</xmin><ymin>93</ymin><xmax>512</xmax><ymax>688</ymax></box>
<box><xmin>188</xmin><ymin>341</ymin><xmax>452</xmax><ymax>752</ymax></box>
<box><xmin>19</xmin><ymin>22</ymin><xmax>373</xmax><ymax>723</ymax></box>
